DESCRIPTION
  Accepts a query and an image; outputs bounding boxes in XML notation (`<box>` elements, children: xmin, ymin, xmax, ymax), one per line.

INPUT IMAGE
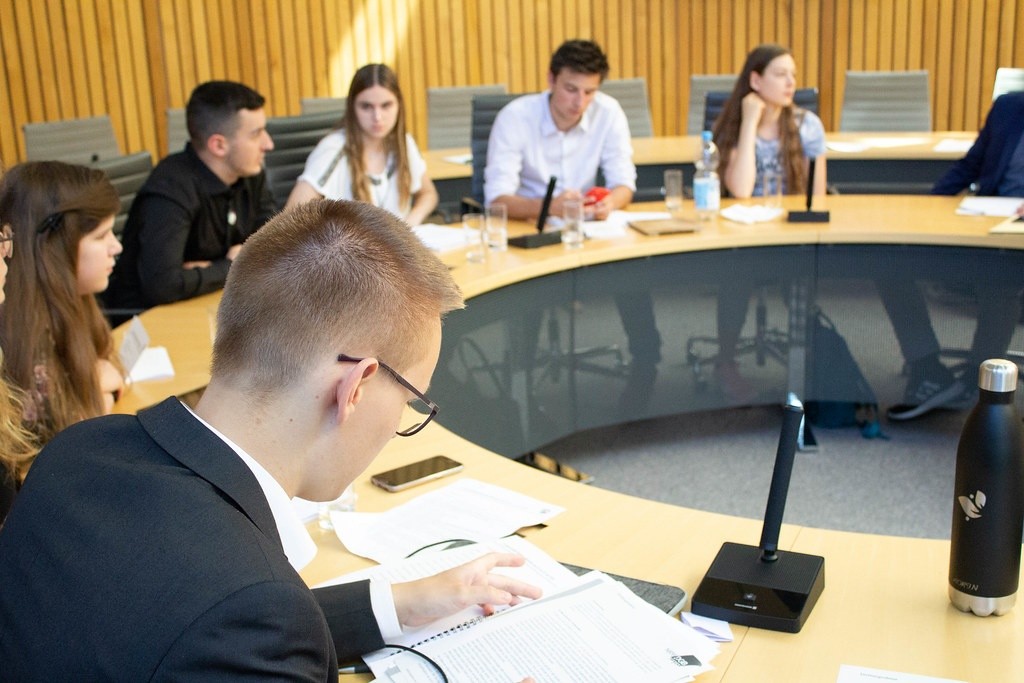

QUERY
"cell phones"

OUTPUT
<box><xmin>370</xmin><ymin>455</ymin><xmax>464</xmax><ymax>492</ymax></box>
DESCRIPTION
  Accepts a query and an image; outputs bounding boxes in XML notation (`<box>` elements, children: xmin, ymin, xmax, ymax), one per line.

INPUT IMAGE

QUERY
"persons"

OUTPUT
<box><xmin>1</xmin><ymin>198</ymin><xmax>542</xmax><ymax>683</ymax></box>
<box><xmin>284</xmin><ymin>63</ymin><xmax>441</xmax><ymax>232</ymax></box>
<box><xmin>879</xmin><ymin>90</ymin><xmax>1024</xmax><ymax>418</ymax></box>
<box><xmin>484</xmin><ymin>39</ymin><xmax>663</xmax><ymax>415</ymax></box>
<box><xmin>104</xmin><ymin>79</ymin><xmax>278</xmax><ymax>329</ymax></box>
<box><xmin>0</xmin><ymin>161</ymin><xmax>124</xmax><ymax>489</ymax></box>
<box><xmin>711</xmin><ymin>43</ymin><xmax>833</xmax><ymax>406</ymax></box>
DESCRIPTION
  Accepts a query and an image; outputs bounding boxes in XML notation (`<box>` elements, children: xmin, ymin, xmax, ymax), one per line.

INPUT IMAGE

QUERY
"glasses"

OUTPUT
<box><xmin>0</xmin><ymin>224</ymin><xmax>14</xmax><ymax>259</ymax></box>
<box><xmin>338</xmin><ymin>354</ymin><xmax>440</xmax><ymax>437</ymax></box>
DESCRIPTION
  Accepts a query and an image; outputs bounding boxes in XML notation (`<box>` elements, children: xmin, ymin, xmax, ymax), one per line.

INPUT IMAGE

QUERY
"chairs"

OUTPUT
<box><xmin>1</xmin><ymin>67</ymin><xmax>1024</xmax><ymax>420</ymax></box>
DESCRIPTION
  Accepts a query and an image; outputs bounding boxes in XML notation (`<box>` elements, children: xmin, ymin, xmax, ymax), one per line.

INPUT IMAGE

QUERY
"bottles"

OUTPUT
<box><xmin>693</xmin><ymin>130</ymin><xmax>721</xmax><ymax>222</ymax></box>
<box><xmin>946</xmin><ymin>356</ymin><xmax>1024</xmax><ymax>617</ymax></box>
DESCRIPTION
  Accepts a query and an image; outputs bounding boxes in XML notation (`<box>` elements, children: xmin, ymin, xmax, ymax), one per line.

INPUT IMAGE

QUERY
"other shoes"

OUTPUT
<box><xmin>618</xmin><ymin>362</ymin><xmax>654</xmax><ymax>410</ymax></box>
<box><xmin>712</xmin><ymin>362</ymin><xmax>759</xmax><ymax>406</ymax></box>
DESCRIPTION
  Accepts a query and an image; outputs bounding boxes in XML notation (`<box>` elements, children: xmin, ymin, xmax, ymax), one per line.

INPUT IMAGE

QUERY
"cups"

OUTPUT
<box><xmin>663</xmin><ymin>169</ymin><xmax>683</xmax><ymax>211</ymax></box>
<box><xmin>462</xmin><ymin>213</ymin><xmax>488</xmax><ymax>263</ymax></box>
<box><xmin>762</xmin><ymin>174</ymin><xmax>783</xmax><ymax>214</ymax></box>
<box><xmin>560</xmin><ymin>199</ymin><xmax>584</xmax><ymax>251</ymax></box>
<box><xmin>484</xmin><ymin>203</ymin><xmax>508</xmax><ymax>254</ymax></box>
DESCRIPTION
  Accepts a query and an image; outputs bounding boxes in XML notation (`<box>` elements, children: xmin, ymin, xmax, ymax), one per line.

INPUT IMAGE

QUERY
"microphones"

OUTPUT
<box><xmin>787</xmin><ymin>157</ymin><xmax>830</xmax><ymax>223</ymax></box>
<box><xmin>690</xmin><ymin>404</ymin><xmax>825</xmax><ymax>633</ymax></box>
<box><xmin>506</xmin><ymin>176</ymin><xmax>562</xmax><ymax>249</ymax></box>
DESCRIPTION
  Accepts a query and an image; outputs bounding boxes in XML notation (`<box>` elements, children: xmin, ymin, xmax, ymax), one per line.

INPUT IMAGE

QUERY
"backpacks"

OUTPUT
<box><xmin>809</xmin><ymin>323</ymin><xmax>877</xmax><ymax>429</ymax></box>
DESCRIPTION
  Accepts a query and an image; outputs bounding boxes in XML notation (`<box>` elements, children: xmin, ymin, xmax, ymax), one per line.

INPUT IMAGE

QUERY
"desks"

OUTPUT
<box><xmin>111</xmin><ymin>195</ymin><xmax>1024</xmax><ymax>681</ymax></box>
<box><xmin>421</xmin><ymin>131</ymin><xmax>980</xmax><ymax>210</ymax></box>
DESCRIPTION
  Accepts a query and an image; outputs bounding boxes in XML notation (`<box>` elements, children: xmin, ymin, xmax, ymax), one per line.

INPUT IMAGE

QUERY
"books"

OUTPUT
<box><xmin>314</xmin><ymin>534</ymin><xmax>720</xmax><ymax>683</ymax></box>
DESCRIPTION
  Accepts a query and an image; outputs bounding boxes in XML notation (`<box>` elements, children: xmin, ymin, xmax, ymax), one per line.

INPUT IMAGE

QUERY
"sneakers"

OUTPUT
<box><xmin>944</xmin><ymin>365</ymin><xmax>984</xmax><ymax>408</ymax></box>
<box><xmin>887</xmin><ymin>370</ymin><xmax>964</xmax><ymax>418</ymax></box>
